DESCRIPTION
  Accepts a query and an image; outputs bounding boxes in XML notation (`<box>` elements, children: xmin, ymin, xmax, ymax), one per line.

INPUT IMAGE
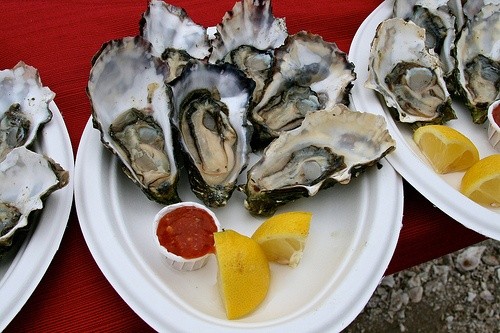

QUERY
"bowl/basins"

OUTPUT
<box><xmin>153</xmin><ymin>201</ymin><xmax>220</xmax><ymax>271</ymax></box>
<box><xmin>487</xmin><ymin>100</ymin><xmax>499</xmax><ymax>154</ymax></box>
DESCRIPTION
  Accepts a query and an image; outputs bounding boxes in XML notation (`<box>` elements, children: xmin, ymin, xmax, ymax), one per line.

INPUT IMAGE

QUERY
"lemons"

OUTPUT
<box><xmin>253</xmin><ymin>212</ymin><xmax>312</xmax><ymax>269</ymax></box>
<box><xmin>214</xmin><ymin>228</ymin><xmax>270</xmax><ymax>320</ymax></box>
<box><xmin>413</xmin><ymin>124</ymin><xmax>479</xmax><ymax>174</ymax></box>
<box><xmin>461</xmin><ymin>153</ymin><xmax>500</xmax><ymax>206</ymax></box>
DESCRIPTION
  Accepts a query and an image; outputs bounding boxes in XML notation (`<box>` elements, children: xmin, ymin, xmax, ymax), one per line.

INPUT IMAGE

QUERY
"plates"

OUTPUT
<box><xmin>73</xmin><ymin>27</ymin><xmax>406</xmax><ymax>333</ymax></box>
<box><xmin>348</xmin><ymin>0</ymin><xmax>500</xmax><ymax>242</ymax></box>
<box><xmin>0</xmin><ymin>99</ymin><xmax>75</xmax><ymax>333</ymax></box>
